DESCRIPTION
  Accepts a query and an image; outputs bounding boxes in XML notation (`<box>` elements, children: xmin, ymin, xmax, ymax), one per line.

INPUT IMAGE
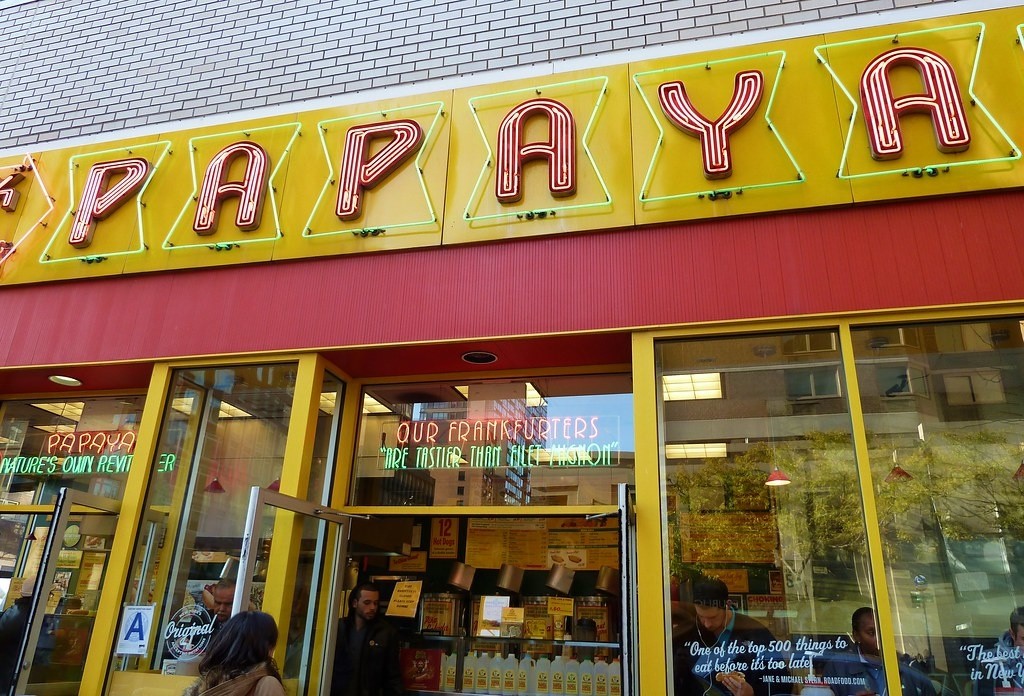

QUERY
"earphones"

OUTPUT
<box><xmin>725</xmin><ymin>604</ymin><xmax>728</xmax><ymax>609</ymax></box>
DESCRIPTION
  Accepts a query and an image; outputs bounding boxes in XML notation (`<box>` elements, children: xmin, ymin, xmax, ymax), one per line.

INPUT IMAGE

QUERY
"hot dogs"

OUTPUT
<box><xmin>715</xmin><ymin>671</ymin><xmax>745</xmax><ymax>681</ymax></box>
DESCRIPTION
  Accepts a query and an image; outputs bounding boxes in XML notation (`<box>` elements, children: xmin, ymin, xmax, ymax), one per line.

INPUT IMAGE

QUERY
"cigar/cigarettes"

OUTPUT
<box><xmin>222</xmin><ymin>615</ymin><xmax>225</xmax><ymax>621</ymax></box>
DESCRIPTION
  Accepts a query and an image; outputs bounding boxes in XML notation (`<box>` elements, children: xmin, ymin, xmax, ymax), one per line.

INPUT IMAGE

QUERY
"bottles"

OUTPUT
<box><xmin>993</xmin><ymin>687</ymin><xmax>1023</xmax><ymax>696</ymax></box>
<box><xmin>800</xmin><ymin>679</ymin><xmax>835</xmax><ymax>696</ymax></box>
<box><xmin>562</xmin><ymin>633</ymin><xmax>572</xmax><ymax>658</ymax></box>
<box><xmin>439</xmin><ymin>650</ymin><xmax>621</xmax><ymax>696</ymax></box>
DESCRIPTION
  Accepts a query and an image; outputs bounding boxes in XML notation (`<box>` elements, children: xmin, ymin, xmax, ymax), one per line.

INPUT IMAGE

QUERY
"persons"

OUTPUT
<box><xmin>973</xmin><ymin>606</ymin><xmax>1024</xmax><ymax>696</ymax></box>
<box><xmin>330</xmin><ymin>583</ymin><xmax>402</xmax><ymax>696</ymax></box>
<box><xmin>178</xmin><ymin>578</ymin><xmax>237</xmax><ymax>652</ymax></box>
<box><xmin>822</xmin><ymin>607</ymin><xmax>938</xmax><ymax>696</ymax></box>
<box><xmin>672</xmin><ymin>576</ymin><xmax>794</xmax><ymax>696</ymax></box>
<box><xmin>182</xmin><ymin>611</ymin><xmax>286</xmax><ymax>696</ymax></box>
<box><xmin>0</xmin><ymin>575</ymin><xmax>49</xmax><ymax>696</ymax></box>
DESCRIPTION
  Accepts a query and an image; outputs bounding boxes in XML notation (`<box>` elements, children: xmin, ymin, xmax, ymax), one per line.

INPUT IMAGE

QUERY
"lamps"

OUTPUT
<box><xmin>495</xmin><ymin>564</ymin><xmax>525</xmax><ymax>593</ymax></box>
<box><xmin>884</xmin><ymin>439</ymin><xmax>914</xmax><ymax>485</ymax></box>
<box><xmin>764</xmin><ymin>410</ymin><xmax>791</xmax><ymax>486</ymax></box>
<box><xmin>447</xmin><ymin>563</ymin><xmax>476</xmax><ymax>592</ymax></box>
<box><xmin>595</xmin><ymin>564</ymin><xmax>622</xmax><ymax>598</ymax></box>
<box><xmin>1012</xmin><ymin>459</ymin><xmax>1024</xmax><ymax>481</ymax></box>
<box><xmin>543</xmin><ymin>563</ymin><xmax>575</xmax><ymax>596</ymax></box>
<box><xmin>202</xmin><ymin>399</ymin><xmax>230</xmax><ymax>493</ymax></box>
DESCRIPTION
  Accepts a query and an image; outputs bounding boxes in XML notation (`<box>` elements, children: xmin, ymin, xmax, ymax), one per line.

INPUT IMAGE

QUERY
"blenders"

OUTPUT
<box><xmin>576</xmin><ymin>619</ymin><xmax>597</xmax><ymax>665</ymax></box>
